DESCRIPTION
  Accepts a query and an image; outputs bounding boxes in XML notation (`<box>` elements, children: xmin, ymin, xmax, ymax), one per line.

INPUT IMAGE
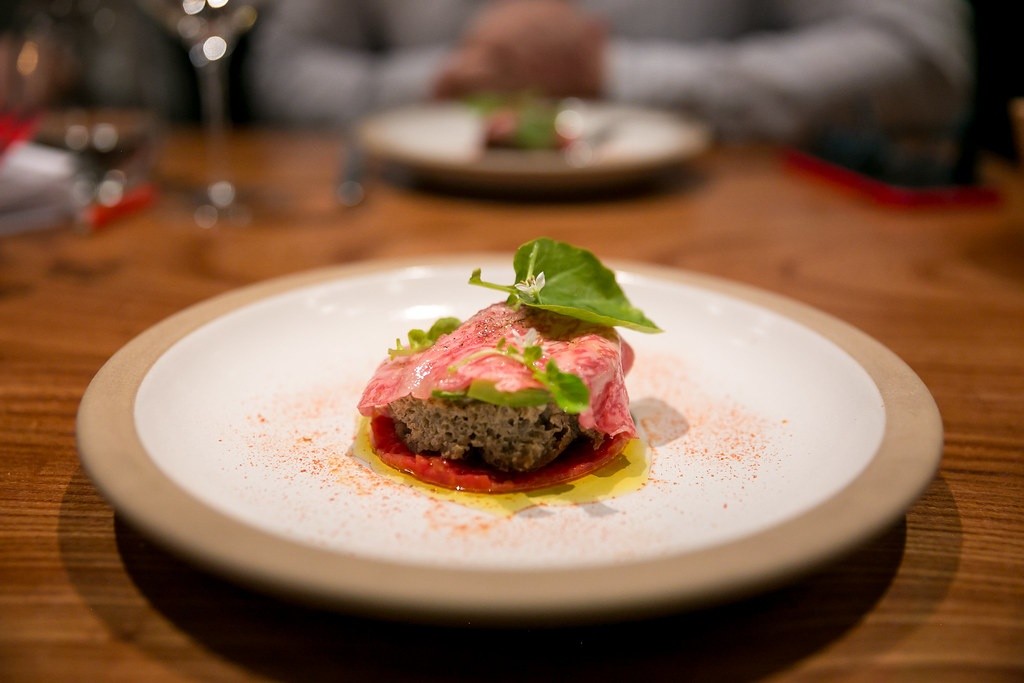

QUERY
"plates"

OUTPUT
<box><xmin>75</xmin><ymin>249</ymin><xmax>946</xmax><ymax>618</ymax></box>
<box><xmin>350</xmin><ymin>95</ymin><xmax>713</xmax><ymax>189</ymax></box>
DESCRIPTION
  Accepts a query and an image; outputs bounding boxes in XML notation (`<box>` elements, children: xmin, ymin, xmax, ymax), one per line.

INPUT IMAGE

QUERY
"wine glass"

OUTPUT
<box><xmin>140</xmin><ymin>0</ymin><xmax>271</xmax><ymax>219</ymax></box>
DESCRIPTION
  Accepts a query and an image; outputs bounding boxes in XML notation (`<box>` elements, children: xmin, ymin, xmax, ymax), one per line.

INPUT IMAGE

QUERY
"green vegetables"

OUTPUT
<box><xmin>387</xmin><ymin>234</ymin><xmax>665</xmax><ymax>414</ymax></box>
<box><xmin>461</xmin><ymin>90</ymin><xmax>569</xmax><ymax>147</ymax></box>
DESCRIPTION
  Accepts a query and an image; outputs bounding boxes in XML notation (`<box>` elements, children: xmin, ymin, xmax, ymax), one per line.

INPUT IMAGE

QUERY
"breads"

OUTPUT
<box><xmin>386</xmin><ymin>393</ymin><xmax>607</xmax><ymax>472</ymax></box>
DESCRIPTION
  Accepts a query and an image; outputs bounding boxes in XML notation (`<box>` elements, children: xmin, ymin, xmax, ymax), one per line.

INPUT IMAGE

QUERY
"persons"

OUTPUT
<box><xmin>246</xmin><ymin>0</ymin><xmax>978</xmax><ymax>129</ymax></box>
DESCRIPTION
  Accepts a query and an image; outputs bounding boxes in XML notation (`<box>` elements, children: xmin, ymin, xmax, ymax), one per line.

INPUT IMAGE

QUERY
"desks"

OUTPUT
<box><xmin>0</xmin><ymin>109</ymin><xmax>1024</xmax><ymax>683</ymax></box>
